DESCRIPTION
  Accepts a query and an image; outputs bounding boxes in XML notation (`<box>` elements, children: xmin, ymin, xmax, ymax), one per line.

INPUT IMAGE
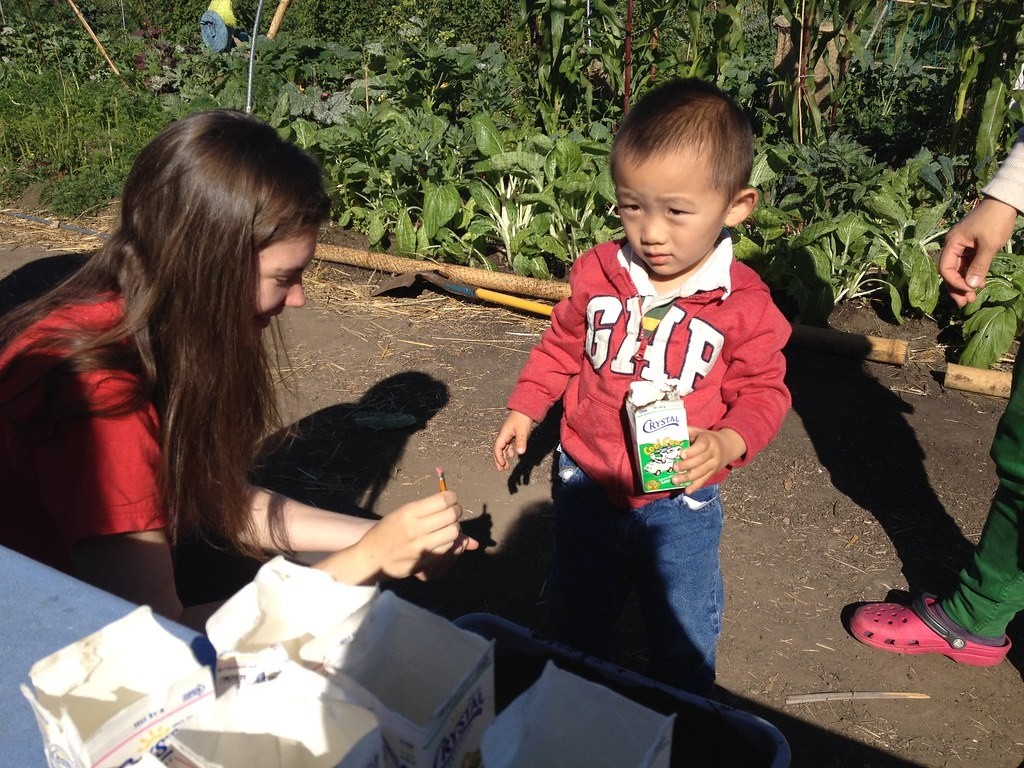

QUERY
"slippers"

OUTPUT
<box><xmin>848</xmin><ymin>592</ymin><xmax>1013</xmax><ymax>667</ymax></box>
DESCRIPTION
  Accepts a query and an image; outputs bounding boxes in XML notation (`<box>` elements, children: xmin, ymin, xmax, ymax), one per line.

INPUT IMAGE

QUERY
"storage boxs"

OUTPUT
<box><xmin>21</xmin><ymin>556</ymin><xmax>793</xmax><ymax>768</ymax></box>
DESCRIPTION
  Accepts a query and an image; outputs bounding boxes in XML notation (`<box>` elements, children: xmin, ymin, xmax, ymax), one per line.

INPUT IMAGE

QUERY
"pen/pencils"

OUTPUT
<box><xmin>435</xmin><ymin>465</ymin><xmax>450</xmax><ymax>495</ymax></box>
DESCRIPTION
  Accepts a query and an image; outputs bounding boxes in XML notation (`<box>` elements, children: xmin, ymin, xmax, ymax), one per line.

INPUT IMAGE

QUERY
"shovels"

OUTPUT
<box><xmin>370</xmin><ymin>266</ymin><xmax>555</xmax><ymax>319</ymax></box>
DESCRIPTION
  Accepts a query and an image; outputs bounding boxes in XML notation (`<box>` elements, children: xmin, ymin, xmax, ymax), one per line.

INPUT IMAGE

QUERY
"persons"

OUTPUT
<box><xmin>1</xmin><ymin>113</ymin><xmax>479</xmax><ymax>634</ymax></box>
<box><xmin>850</xmin><ymin>139</ymin><xmax>1024</xmax><ymax>668</ymax></box>
<box><xmin>494</xmin><ymin>79</ymin><xmax>792</xmax><ymax>691</ymax></box>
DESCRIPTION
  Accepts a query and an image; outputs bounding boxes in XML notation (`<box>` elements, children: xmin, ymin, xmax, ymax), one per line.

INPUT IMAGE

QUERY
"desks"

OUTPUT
<box><xmin>0</xmin><ymin>547</ymin><xmax>202</xmax><ymax>768</ymax></box>
